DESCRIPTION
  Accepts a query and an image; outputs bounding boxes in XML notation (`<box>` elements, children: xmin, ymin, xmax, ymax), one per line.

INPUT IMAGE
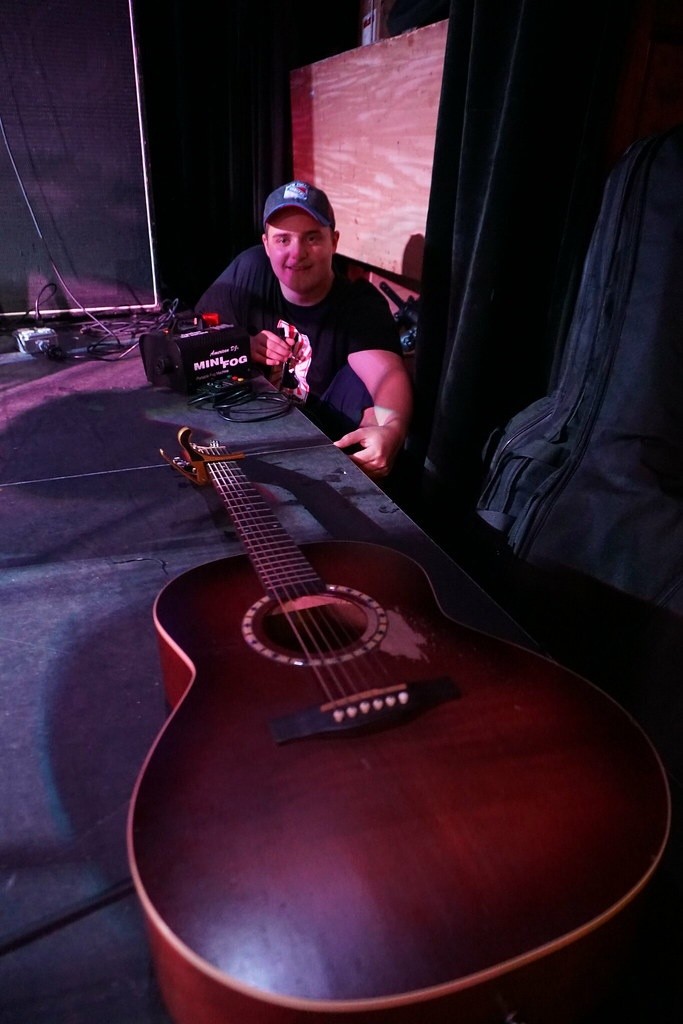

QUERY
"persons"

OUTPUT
<box><xmin>195</xmin><ymin>182</ymin><xmax>412</xmax><ymax>480</ymax></box>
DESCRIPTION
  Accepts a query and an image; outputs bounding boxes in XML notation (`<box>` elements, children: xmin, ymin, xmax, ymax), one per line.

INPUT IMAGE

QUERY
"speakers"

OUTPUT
<box><xmin>0</xmin><ymin>0</ymin><xmax>161</xmax><ymax>316</ymax></box>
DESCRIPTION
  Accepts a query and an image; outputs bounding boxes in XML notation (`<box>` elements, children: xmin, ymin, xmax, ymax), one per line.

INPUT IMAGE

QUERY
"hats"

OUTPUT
<box><xmin>263</xmin><ymin>180</ymin><xmax>336</xmax><ymax>234</ymax></box>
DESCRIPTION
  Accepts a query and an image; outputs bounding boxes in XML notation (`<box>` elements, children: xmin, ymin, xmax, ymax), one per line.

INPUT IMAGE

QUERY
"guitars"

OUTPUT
<box><xmin>124</xmin><ymin>421</ymin><xmax>673</xmax><ymax>1024</ymax></box>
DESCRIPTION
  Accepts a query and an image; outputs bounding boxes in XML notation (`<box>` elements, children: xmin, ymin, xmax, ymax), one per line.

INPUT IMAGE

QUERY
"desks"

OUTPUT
<box><xmin>0</xmin><ymin>311</ymin><xmax>683</xmax><ymax>1024</ymax></box>
